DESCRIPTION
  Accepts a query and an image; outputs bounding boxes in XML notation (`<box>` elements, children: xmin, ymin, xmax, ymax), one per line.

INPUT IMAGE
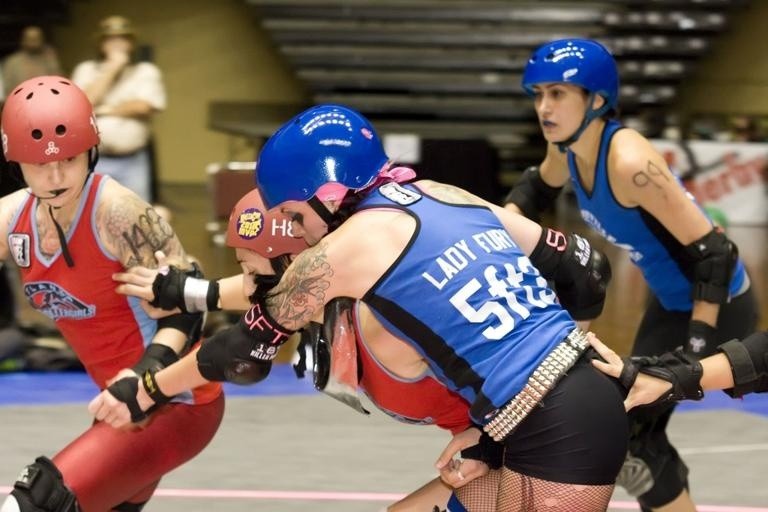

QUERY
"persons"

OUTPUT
<box><xmin>68</xmin><ymin>14</ymin><xmax>167</xmax><ymax>205</ymax></box>
<box><xmin>87</xmin><ymin>186</ymin><xmax>491</xmax><ymax>511</ymax></box>
<box><xmin>502</xmin><ymin>38</ymin><xmax>757</xmax><ymax>511</ymax></box>
<box><xmin>0</xmin><ymin>22</ymin><xmax>63</xmax><ymax>106</ymax></box>
<box><xmin>583</xmin><ymin>328</ymin><xmax>767</xmax><ymax>414</ymax></box>
<box><xmin>1</xmin><ymin>74</ymin><xmax>227</xmax><ymax>511</ymax></box>
<box><xmin>88</xmin><ymin>104</ymin><xmax>631</xmax><ymax>510</ymax></box>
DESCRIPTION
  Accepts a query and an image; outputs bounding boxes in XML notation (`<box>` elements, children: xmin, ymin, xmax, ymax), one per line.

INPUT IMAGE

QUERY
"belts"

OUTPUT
<box><xmin>481</xmin><ymin>328</ymin><xmax>589</xmax><ymax>442</ymax></box>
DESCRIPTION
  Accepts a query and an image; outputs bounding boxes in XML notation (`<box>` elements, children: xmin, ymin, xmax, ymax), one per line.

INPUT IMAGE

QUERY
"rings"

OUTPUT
<box><xmin>456</xmin><ymin>470</ymin><xmax>465</xmax><ymax>481</ymax></box>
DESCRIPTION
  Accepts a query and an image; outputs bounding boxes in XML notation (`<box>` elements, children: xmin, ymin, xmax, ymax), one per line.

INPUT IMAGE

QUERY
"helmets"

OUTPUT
<box><xmin>521</xmin><ymin>37</ymin><xmax>619</xmax><ymax>115</ymax></box>
<box><xmin>225</xmin><ymin>188</ymin><xmax>308</xmax><ymax>259</ymax></box>
<box><xmin>255</xmin><ymin>104</ymin><xmax>392</xmax><ymax>239</ymax></box>
<box><xmin>98</xmin><ymin>15</ymin><xmax>139</xmax><ymax>40</ymax></box>
<box><xmin>2</xmin><ymin>74</ymin><xmax>101</xmax><ymax>163</ymax></box>
